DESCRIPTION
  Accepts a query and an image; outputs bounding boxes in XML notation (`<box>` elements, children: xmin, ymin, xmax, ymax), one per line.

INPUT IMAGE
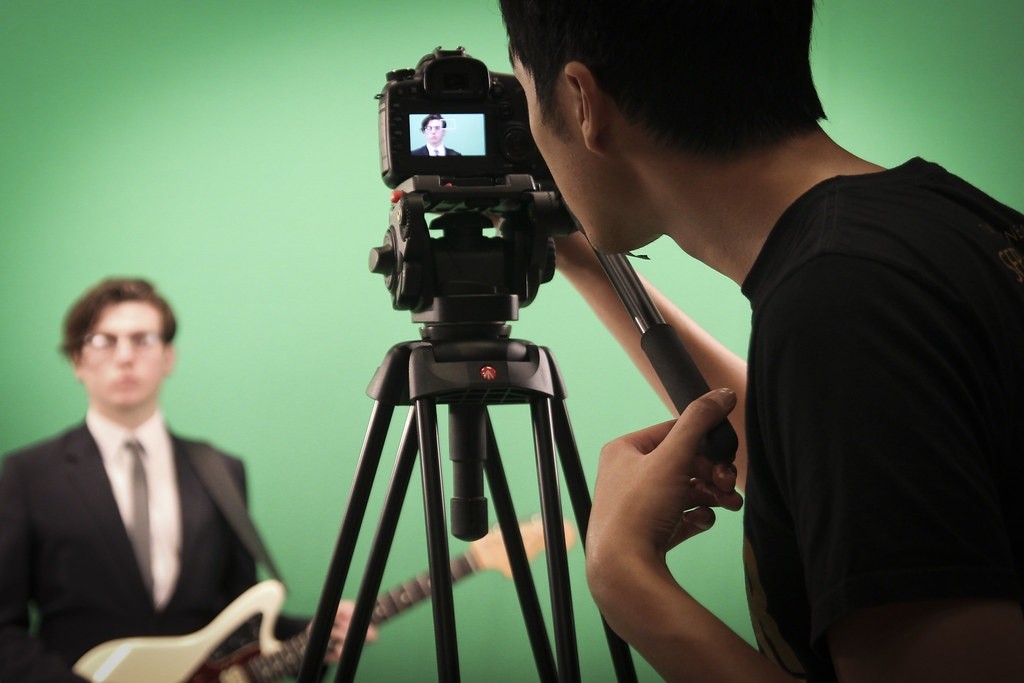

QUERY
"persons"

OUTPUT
<box><xmin>501</xmin><ymin>0</ymin><xmax>1024</xmax><ymax>683</ymax></box>
<box><xmin>0</xmin><ymin>274</ymin><xmax>377</xmax><ymax>683</ymax></box>
<box><xmin>410</xmin><ymin>114</ymin><xmax>461</xmax><ymax>156</ymax></box>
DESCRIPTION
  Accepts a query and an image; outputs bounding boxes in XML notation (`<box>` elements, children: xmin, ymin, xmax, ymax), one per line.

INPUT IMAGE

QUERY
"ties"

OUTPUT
<box><xmin>434</xmin><ymin>149</ymin><xmax>439</xmax><ymax>156</ymax></box>
<box><xmin>126</xmin><ymin>439</ymin><xmax>153</xmax><ymax>605</ymax></box>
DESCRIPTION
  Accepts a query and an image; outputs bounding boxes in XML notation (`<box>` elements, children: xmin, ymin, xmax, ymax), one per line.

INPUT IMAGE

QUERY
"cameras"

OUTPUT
<box><xmin>374</xmin><ymin>46</ymin><xmax>553</xmax><ymax>187</ymax></box>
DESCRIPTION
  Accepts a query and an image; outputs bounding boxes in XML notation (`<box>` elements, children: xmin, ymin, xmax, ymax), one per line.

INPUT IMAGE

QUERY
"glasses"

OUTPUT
<box><xmin>424</xmin><ymin>126</ymin><xmax>442</xmax><ymax>131</ymax></box>
<box><xmin>83</xmin><ymin>329</ymin><xmax>164</xmax><ymax>351</ymax></box>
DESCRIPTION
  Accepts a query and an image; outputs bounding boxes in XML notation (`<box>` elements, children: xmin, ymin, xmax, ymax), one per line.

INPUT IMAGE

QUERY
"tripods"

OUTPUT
<box><xmin>299</xmin><ymin>176</ymin><xmax>740</xmax><ymax>683</ymax></box>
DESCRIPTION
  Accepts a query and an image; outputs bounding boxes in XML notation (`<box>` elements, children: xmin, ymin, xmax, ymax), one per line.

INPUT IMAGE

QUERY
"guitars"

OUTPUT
<box><xmin>72</xmin><ymin>511</ymin><xmax>575</xmax><ymax>683</ymax></box>
